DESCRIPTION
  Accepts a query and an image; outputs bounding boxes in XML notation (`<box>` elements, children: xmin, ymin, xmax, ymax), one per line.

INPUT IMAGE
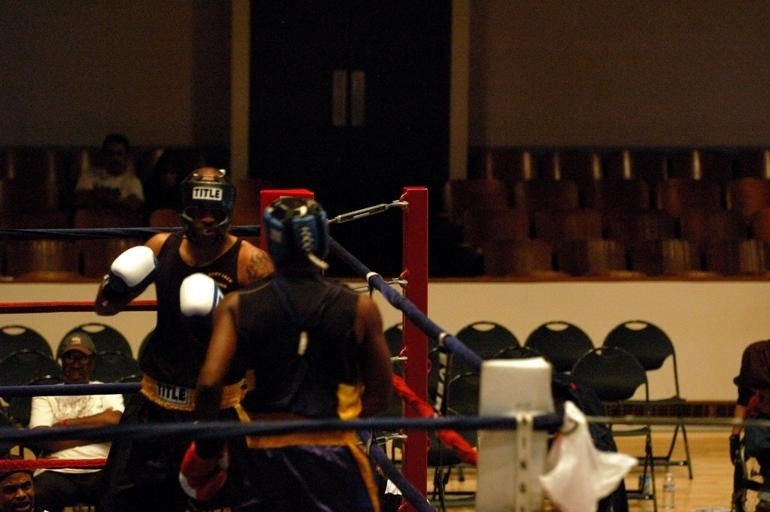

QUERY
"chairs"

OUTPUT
<box><xmin>679</xmin><ymin>206</ymin><xmax>747</xmax><ymax>259</ymax></box>
<box><xmin>481</xmin><ymin>149</ymin><xmax>537</xmax><ymax>182</ymax></box>
<box><xmin>533</xmin><ymin>208</ymin><xmax>602</xmax><ymax>266</ymax></box>
<box><xmin>609</xmin><ymin>208</ymin><xmax>675</xmax><ymax>268</ymax></box>
<box><xmin>750</xmin><ymin>209</ymin><xmax>769</xmax><ymax>240</ymax></box>
<box><xmin>656</xmin><ymin>178</ymin><xmax>721</xmax><ymax>228</ymax></box>
<box><xmin>691</xmin><ymin>149</ymin><xmax>732</xmax><ymax>180</ymax></box>
<box><xmin>485</xmin><ymin>238</ymin><xmax>554</xmax><ymax>274</ymax></box>
<box><xmin>1</xmin><ymin>147</ymin><xmax>59</xmax><ymax>206</ymax></box>
<box><xmin>76</xmin><ymin>146</ymin><xmax>103</xmax><ymax>174</ymax></box>
<box><xmin>75</xmin><ymin>209</ymin><xmax>149</xmax><ymax>229</ymax></box>
<box><xmin>708</xmin><ymin>240</ymin><xmax>770</xmax><ymax>274</ymax></box>
<box><xmin>431</xmin><ymin>320</ymin><xmax>523</xmax><ymax>483</ymax></box>
<box><xmin>115</xmin><ymin>374</ymin><xmax>145</xmax><ymax>384</ymax></box>
<box><xmin>428</xmin><ymin>443</ymin><xmax>475</xmax><ymax>511</ymax></box>
<box><xmin>443</xmin><ymin>178</ymin><xmax>509</xmax><ymax>226</ymax></box>
<box><xmin>0</xmin><ymin>326</ymin><xmax>54</xmax><ymax>365</ymax></box>
<box><xmin>0</xmin><ymin>207</ymin><xmax>65</xmax><ymax>229</ymax></box>
<box><xmin>601</xmin><ymin>319</ymin><xmax>694</xmax><ymax>481</ymax></box>
<box><xmin>570</xmin><ymin>346</ymin><xmax>657</xmax><ymax>512</ymax></box>
<box><xmin>84</xmin><ymin>239</ymin><xmax>149</xmax><ymax>280</ymax></box>
<box><xmin>522</xmin><ymin>321</ymin><xmax>593</xmax><ymax>375</ymax></box>
<box><xmin>635</xmin><ymin>240</ymin><xmax>704</xmax><ymax>272</ymax></box>
<box><xmin>582</xmin><ymin>178</ymin><xmax>650</xmax><ymax>235</ymax></box>
<box><xmin>57</xmin><ymin>321</ymin><xmax>134</xmax><ymax>379</ymax></box>
<box><xmin>91</xmin><ymin>350</ymin><xmax>140</xmax><ymax>383</ymax></box>
<box><xmin>3</xmin><ymin>238</ymin><xmax>79</xmax><ymax>278</ymax></box>
<box><xmin>491</xmin><ymin>347</ymin><xmax>545</xmax><ymax>360</ymax></box>
<box><xmin>540</xmin><ymin>146</ymin><xmax>603</xmax><ymax>180</ymax></box>
<box><xmin>512</xmin><ymin>180</ymin><xmax>579</xmax><ymax>211</ymax></box>
<box><xmin>386</xmin><ymin>322</ymin><xmax>403</xmax><ymax>378</ymax></box>
<box><xmin>725</xmin><ymin>177</ymin><xmax>770</xmax><ymax>221</ymax></box>
<box><xmin>557</xmin><ymin>238</ymin><xmax>627</xmax><ymax>274</ymax></box>
<box><xmin>620</xmin><ymin>149</ymin><xmax>668</xmax><ymax>181</ymax></box>
<box><xmin>1</xmin><ymin>349</ymin><xmax>61</xmax><ymax>386</ymax></box>
<box><xmin>17</xmin><ymin>374</ymin><xmax>67</xmax><ymax>386</ymax></box>
<box><xmin>463</xmin><ymin>207</ymin><xmax>528</xmax><ymax>270</ymax></box>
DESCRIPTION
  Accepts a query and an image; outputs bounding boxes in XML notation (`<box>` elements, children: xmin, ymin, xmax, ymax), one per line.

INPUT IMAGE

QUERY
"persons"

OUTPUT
<box><xmin>0</xmin><ymin>457</ymin><xmax>36</xmax><ymax>512</ymax></box>
<box><xmin>31</xmin><ymin>330</ymin><xmax>128</xmax><ymax>512</ymax></box>
<box><xmin>95</xmin><ymin>167</ymin><xmax>276</xmax><ymax>511</ymax></box>
<box><xmin>68</xmin><ymin>135</ymin><xmax>146</xmax><ymax>225</ymax></box>
<box><xmin>179</xmin><ymin>196</ymin><xmax>393</xmax><ymax>512</ymax></box>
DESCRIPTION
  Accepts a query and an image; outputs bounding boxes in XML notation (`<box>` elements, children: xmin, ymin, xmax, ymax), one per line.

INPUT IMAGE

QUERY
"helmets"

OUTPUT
<box><xmin>179</xmin><ymin>168</ymin><xmax>235</xmax><ymax>239</ymax></box>
<box><xmin>266</xmin><ymin>197</ymin><xmax>329</xmax><ymax>274</ymax></box>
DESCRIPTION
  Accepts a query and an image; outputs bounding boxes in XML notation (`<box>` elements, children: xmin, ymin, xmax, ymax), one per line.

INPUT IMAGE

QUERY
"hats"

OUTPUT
<box><xmin>59</xmin><ymin>333</ymin><xmax>97</xmax><ymax>357</ymax></box>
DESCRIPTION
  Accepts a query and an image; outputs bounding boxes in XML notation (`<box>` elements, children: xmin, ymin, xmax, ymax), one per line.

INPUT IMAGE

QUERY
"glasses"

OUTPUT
<box><xmin>63</xmin><ymin>354</ymin><xmax>92</xmax><ymax>366</ymax></box>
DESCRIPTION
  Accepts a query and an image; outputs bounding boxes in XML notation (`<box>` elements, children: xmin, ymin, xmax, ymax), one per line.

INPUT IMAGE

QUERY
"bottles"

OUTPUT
<box><xmin>662</xmin><ymin>473</ymin><xmax>675</xmax><ymax>508</ymax></box>
<box><xmin>638</xmin><ymin>474</ymin><xmax>650</xmax><ymax>499</ymax></box>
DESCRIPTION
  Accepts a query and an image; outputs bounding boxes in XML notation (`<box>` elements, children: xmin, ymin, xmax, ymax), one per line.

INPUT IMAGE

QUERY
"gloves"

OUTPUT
<box><xmin>730</xmin><ymin>435</ymin><xmax>743</xmax><ymax>464</ymax></box>
<box><xmin>162</xmin><ymin>272</ymin><xmax>225</xmax><ymax>334</ymax></box>
<box><xmin>102</xmin><ymin>247</ymin><xmax>158</xmax><ymax>305</ymax></box>
<box><xmin>178</xmin><ymin>434</ymin><xmax>229</xmax><ymax>499</ymax></box>
